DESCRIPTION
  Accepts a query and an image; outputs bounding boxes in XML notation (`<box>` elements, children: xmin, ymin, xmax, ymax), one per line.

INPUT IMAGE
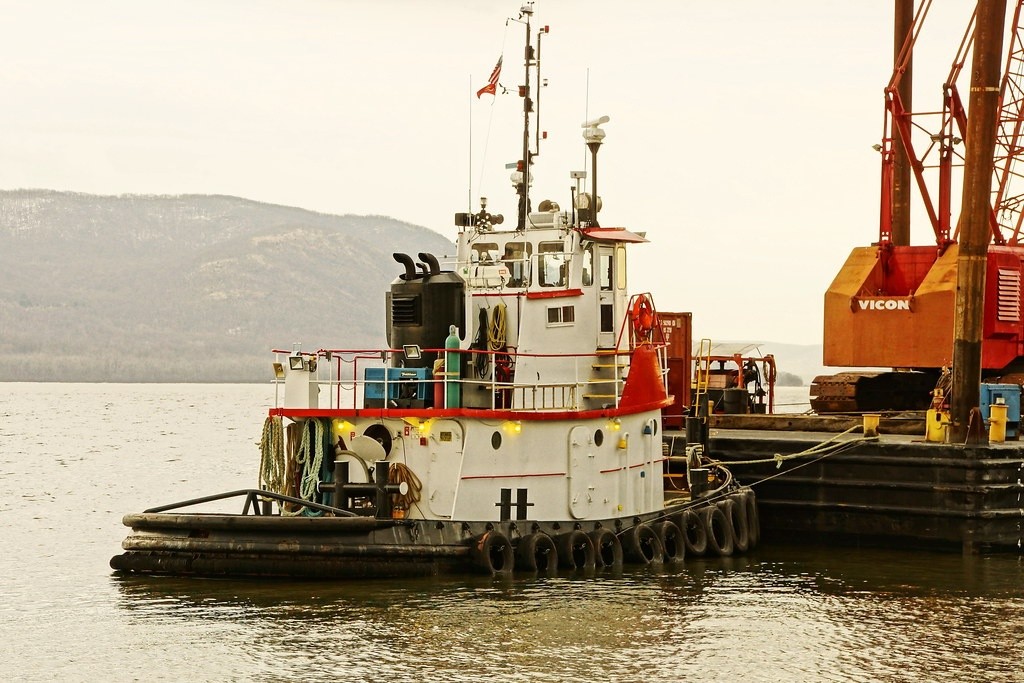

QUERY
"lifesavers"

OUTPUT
<box><xmin>632</xmin><ymin>294</ymin><xmax>654</xmax><ymax>339</ymax></box>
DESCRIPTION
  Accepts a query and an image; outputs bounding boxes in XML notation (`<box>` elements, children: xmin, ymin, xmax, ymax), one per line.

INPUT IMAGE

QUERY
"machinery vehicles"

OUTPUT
<box><xmin>809</xmin><ymin>1</ymin><xmax>1024</xmax><ymax>425</ymax></box>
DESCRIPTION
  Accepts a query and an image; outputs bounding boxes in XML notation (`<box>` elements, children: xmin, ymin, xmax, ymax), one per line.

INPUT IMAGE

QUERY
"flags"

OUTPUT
<box><xmin>475</xmin><ymin>55</ymin><xmax>502</xmax><ymax>100</ymax></box>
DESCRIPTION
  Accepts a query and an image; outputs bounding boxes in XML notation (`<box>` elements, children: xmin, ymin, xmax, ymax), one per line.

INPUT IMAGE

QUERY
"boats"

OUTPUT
<box><xmin>111</xmin><ymin>0</ymin><xmax>761</xmax><ymax>588</ymax></box>
